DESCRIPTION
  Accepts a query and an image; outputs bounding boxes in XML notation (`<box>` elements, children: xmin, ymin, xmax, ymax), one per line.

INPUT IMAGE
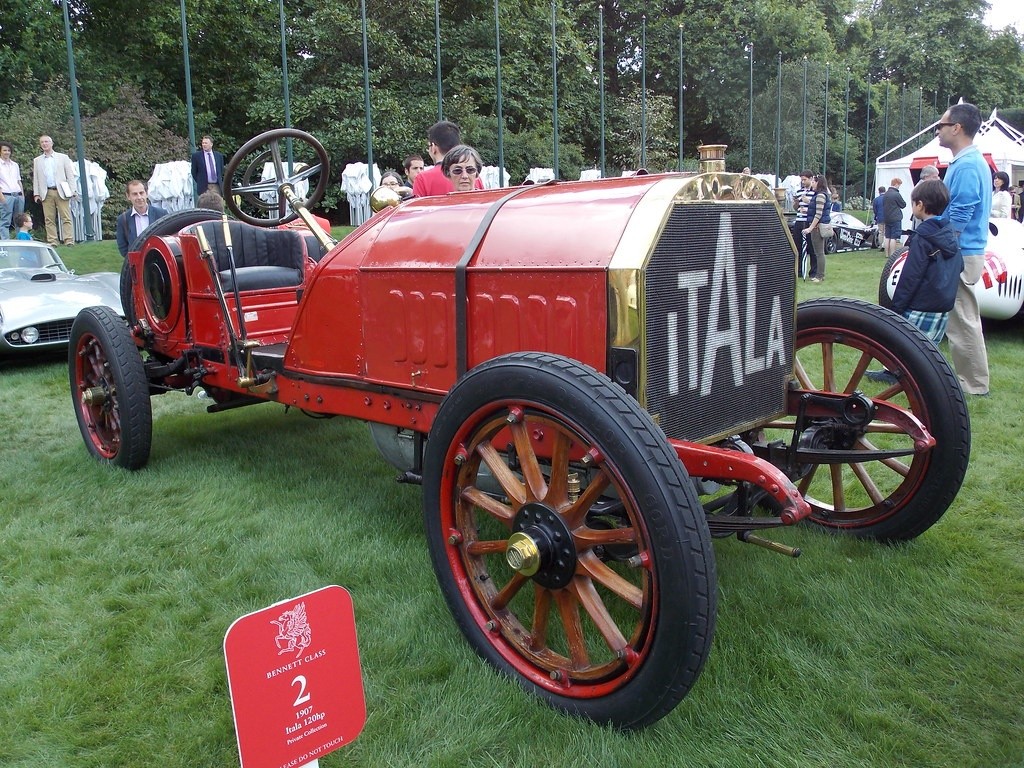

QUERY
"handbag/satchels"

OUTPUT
<box><xmin>819</xmin><ymin>223</ymin><xmax>835</xmax><ymax>238</ymax></box>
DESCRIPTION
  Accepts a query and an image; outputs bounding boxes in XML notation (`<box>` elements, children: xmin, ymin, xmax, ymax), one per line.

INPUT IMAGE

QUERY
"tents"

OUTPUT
<box><xmin>875</xmin><ymin>97</ymin><xmax>1024</xmax><ymax>246</ymax></box>
<box><xmin>149</xmin><ymin>161</ymin><xmax>197</xmax><ymax>216</ymax></box>
<box><xmin>341</xmin><ymin>162</ymin><xmax>382</xmax><ymax>226</ymax></box>
<box><xmin>752</xmin><ymin>169</ymin><xmax>801</xmax><ymax>211</ymax></box>
<box><xmin>478</xmin><ymin>165</ymin><xmax>510</xmax><ymax>190</ymax></box>
<box><xmin>259</xmin><ymin>162</ymin><xmax>310</xmax><ymax>220</ymax></box>
<box><xmin>577</xmin><ymin>170</ymin><xmax>603</xmax><ymax>181</ymax></box>
<box><xmin>525</xmin><ymin>168</ymin><xmax>555</xmax><ymax>184</ymax></box>
<box><xmin>58</xmin><ymin>158</ymin><xmax>111</xmax><ymax>242</ymax></box>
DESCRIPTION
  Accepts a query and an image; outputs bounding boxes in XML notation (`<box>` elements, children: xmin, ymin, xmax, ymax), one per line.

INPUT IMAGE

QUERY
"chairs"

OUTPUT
<box><xmin>303</xmin><ymin>232</ymin><xmax>338</xmax><ymax>263</ymax></box>
<box><xmin>179</xmin><ymin>218</ymin><xmax>306</xmax><ymax>350</ymax></box>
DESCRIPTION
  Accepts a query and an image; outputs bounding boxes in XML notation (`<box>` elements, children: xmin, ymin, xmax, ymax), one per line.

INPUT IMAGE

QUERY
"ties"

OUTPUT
<box><xmin>207</xmin><ymin>153</ymin><xmax>216</xmax><ymax>180</ymax></box>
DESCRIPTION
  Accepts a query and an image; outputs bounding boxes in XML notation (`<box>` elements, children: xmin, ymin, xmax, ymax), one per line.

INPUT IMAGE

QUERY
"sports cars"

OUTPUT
<box><xmin>0</xmin><ymin>239</ymin><xmax>130</xmax><ymax>360</ymax></box>
<box><xmin>879</xmin><ymin>216</ymin><xmax>1024</xmax><ymax>323</ymax></box>
<box><xmin>786</xmin><ymin>211</ymin><xmax>883</xmax><ymax>254</ymax></box>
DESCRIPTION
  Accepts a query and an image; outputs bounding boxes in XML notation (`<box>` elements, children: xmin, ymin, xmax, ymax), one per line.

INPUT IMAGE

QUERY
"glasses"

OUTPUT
<box><xmin>449</xmin><ymin>168</ymin><xmax>477</xmax><ymax>174</ymax></box>
<box><xmin>381</xmin><ymin>182</ymin><xmax>400</xmax><ymax>186</ymax></box>
<box><xmin>25</xmin><ymin>221</ymin><xmax>32</xmax><ymax>223</ymax></box>
<box><xmin>935</xmin><ymin>123</ymin><xmax>963</xmax><ymax>130</ymax></box>
<box><xmin>425</xmin><ymin>142</ymin><xmax>439</xmax><ymax>149</ymax></box>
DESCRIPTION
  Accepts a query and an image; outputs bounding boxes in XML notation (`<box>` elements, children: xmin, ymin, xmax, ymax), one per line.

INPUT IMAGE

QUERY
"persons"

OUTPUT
<box><xmin>441</xmin><ymin>145</ymin><xmax>482</xmax><ymax>191</ymax></box>
<box><xmin>14</xmin><ymin>135</ymin><xmax>77</xmax><ymax>248</ymax></box>
<box><xmin>194</xmin><ymin>192</ymin><xmax>225</xmax><ymax>213</ymax></box>
<box><xmin>0</xmin><ymin>141</ymin><xmax>25</xmax><ymax>240</ymax></box>
<box><xmin>413</xmin><ymin>121</ymin><xmax>485</xmax><ymax>196</ymax></box>
<box><xmin>991</xmin><ymin>171</ymin><xmax>1024</xmax><ymax>224</ymax></box>
<box><xmin>912</xmin><ymin>103</ymin><xmax>993</xmax><ymax>394</ymax></box>
<box><xmin>873</xmin><ymin>178</ymin><xmax>907</xmax><ymax>257</ymax></box>
<box><xmin>864</xmin><ymin>179</ymin><xmax>964</xmax><ymax>385</ymax></box>
<box><xmin>379</xmin><ymin>153</ymin><xmax>424</xmax><ymax>200</ymax></box>
<box><xmin>793</xmin><ymin>170</ymin><xmax>831</xmax><ymax>282</ymax></box>
<box><xmin>191</xmin><ymin>136</ymin><xmax>224</xmax><ymax>201</ymax></box>
<box><xmin>116</xmin><ymin>180</ymin><xmax>169</xmax><ymax>257</ymax></box>
<box><xmin>830</xmin><ymin>194</ymin><xmax>842</xmax><ymax>212</ymax></box>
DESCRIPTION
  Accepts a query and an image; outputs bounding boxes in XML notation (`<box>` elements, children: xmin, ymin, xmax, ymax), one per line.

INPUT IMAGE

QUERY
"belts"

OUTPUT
<box><xmin>48</xmin><ymin>186</ymin><xmax>57</xmax><ymax>190</ymax></box>
<box><xmin>2</xmin><ymin>192</ymin><xmax>22</xmax><ymax>196</ymax></box>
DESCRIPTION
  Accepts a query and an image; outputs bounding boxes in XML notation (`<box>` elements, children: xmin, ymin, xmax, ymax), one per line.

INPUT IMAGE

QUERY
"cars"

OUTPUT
<box><xmin>67</xmin><ymin>125</ymin><xmax>972</xmax><ymax>732</ymax></box>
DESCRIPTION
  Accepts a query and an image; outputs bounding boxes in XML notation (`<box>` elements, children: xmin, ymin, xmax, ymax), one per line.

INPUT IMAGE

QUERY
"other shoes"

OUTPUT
<box><xmin>51</xmin><ymin>245</ymin><xmax>57</xmax><ymax>248</ymax></box>
<box><xmin>864</xmin><ymin>369</ymin><xmax>899</xmax><ymax>385</ymax></box>
<box><xmin>811</xmin><ymin>277</ymin><xmax>823</xmax><ymax>282</ymax></box>
<box><xmin>67</xmin><ymin>243</ymin><xmax>74</xmax><ymax>247</ymax></box>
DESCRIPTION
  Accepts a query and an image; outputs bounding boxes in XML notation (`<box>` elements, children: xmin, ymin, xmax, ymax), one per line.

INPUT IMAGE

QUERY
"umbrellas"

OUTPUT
<box><xmin>802</xmin><ymin>234</ymin><xmax>807</xmax><ymax>282</ymax></box>
